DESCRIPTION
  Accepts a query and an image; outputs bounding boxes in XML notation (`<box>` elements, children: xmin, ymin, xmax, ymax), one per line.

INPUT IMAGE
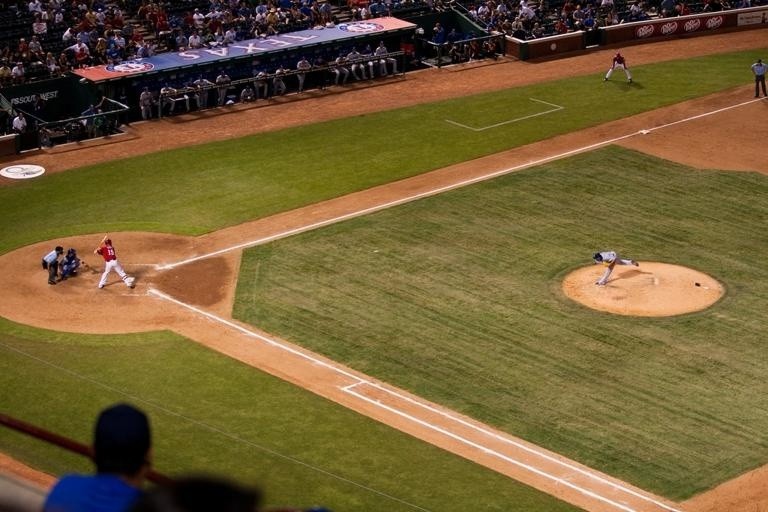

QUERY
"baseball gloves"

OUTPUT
<box><xmin>603</xmin><ymin>260</ymin><xmax>611</xmax><ymax>267</ymax></box>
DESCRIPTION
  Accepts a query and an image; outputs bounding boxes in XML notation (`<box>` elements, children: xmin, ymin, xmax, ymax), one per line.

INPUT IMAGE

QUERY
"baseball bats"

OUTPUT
<box><xmin>94</xmin><ymin>236</ymin><xmax>107</xmax><ymax>255</ymax></box>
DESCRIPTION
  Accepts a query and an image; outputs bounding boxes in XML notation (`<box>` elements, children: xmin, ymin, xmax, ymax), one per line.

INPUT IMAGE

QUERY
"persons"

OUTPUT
<box><xmin>751</xmin><ymin>59</ymin><xmax>767</xmax><ymax>99</ymax></box>
<box><xmin>59</xmin><ymin>247</ymin><xmax>85</xmax><ymax>279</ymax></box>
<box><xmin>591</xmin><ymin>248</ymin><xmax>640</xmax><ymax>287</ymax></box>
<box><xmin>416</xmin><ymin>0</ymin><xmax>768</xmax><ymax>60</ymax></box>
<box><xmin>602</xmin><ymin>48</ymin><xmax>633</xmax><ymax>85</ymax></box>
<box><xmin>0</xmin><ymin>0</ymin><xmax>416</xmax><ymax>149</ymax></box>
<box><xmin>41</xmin><ymin>402</ymin><xmax>150</xmax><ymax>510</ymax></box>
<box><xmin>93</xmin><ymin>239</ymin><xmax>136</xmax><ymax>289</ymax></box>
<box><xmin>43</xmin><ymin>245</ymin><xmax>64</xmax><ymax>286</ymax></box>
<box><xmin>123</xmin><ymin>468</ymin><xmax>264</xmax><ymax>512</ymax></box>
